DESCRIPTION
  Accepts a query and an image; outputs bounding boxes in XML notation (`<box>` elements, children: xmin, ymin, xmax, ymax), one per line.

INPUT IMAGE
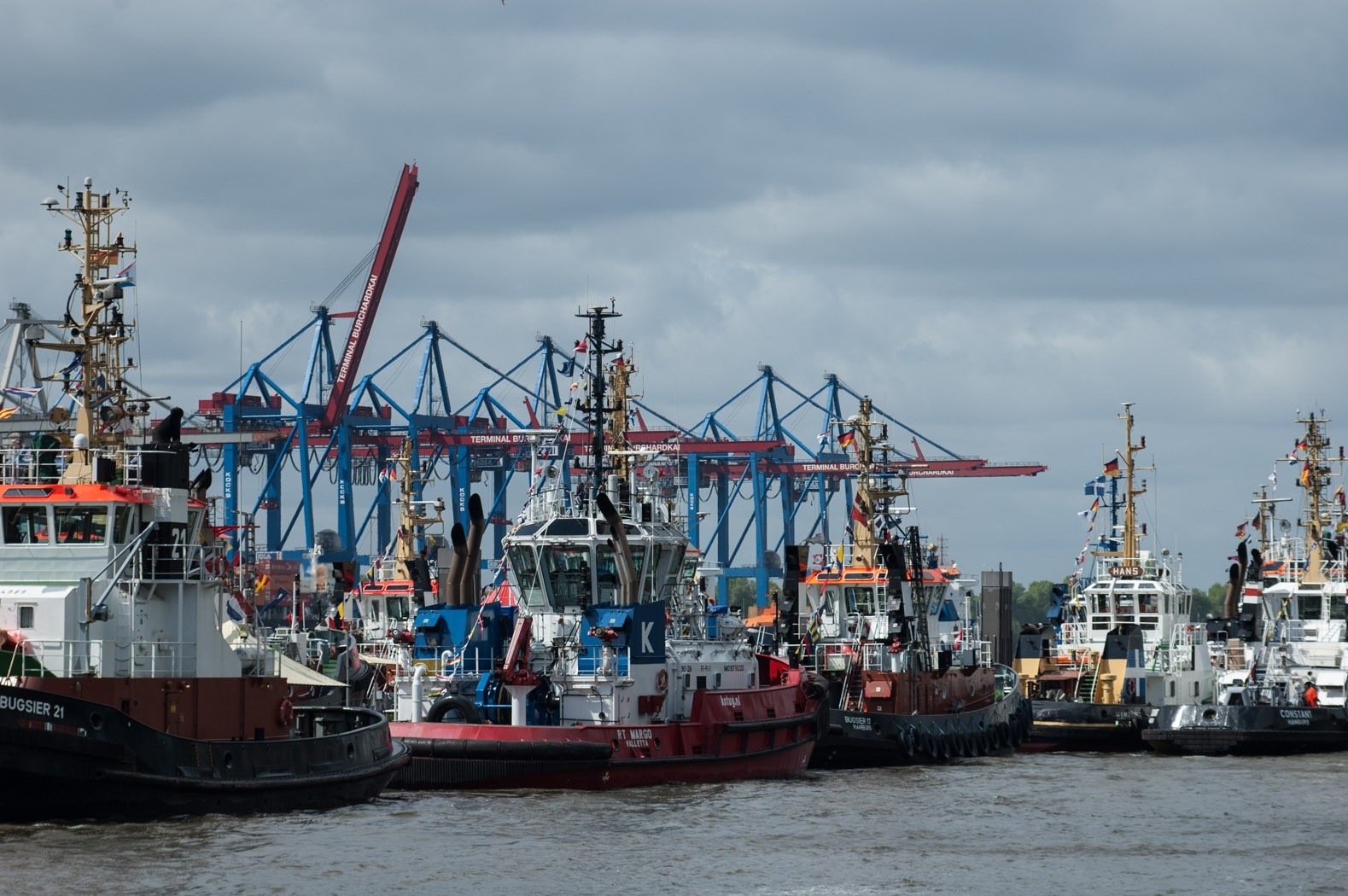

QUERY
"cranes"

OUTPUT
<box><xmin>175</xmin><ymin>168</ymin><xmax>1044</xmax><ymax>632</ymax></box>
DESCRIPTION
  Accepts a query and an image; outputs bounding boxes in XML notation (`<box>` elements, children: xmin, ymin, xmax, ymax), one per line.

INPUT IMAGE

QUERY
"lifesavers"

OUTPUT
<box><xmin>894</xmin><ymin>697</ymin><xmax>1033</xmax><ymax>764</ymax></box>
<box><xmin>220</xmin><ymin>751</ymin><xmax>235</xmax><ymax>771</ymax></box>
<box><xmin>426</xmin><ymin>694</ymin><xmax>483</xmax><ymax>725</ymax></box>
<box><xmin>346</xmin><ymin>742</ymin><xmax>355</xmax><ymax>759</ymax></box>
<box><xmin>88</xmin><ymin>711</ymin><xmax>104</xmax><ymax>732</ymax></box>
<box><xmin>278</xmin><ymin>699</ymin><xmax>294</xmax><ymax>727</ymax></box>
<box><xmin>215</xmin><ymin>554</ymin><xmax>230</xmax><ymax>578</ymax></box>
<box><xmin>1127</xmin><ymin>679</ymin><xmax>1135</xmax><ymax>695</ymax></box>
<box><xmin>656</xmin><ymin>669</ymin><xmax>669</xmax><ymax>690</ymax></box>
<box><xmin>1185</xmin><ymin>625</ymin><xmax>1192</xmax><ymax>642</ymax></box>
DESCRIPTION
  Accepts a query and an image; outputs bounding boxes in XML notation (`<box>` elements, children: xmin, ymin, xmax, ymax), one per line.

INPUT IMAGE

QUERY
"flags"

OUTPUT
<box><xmin>793</xmin><ymin>427</ymin><xmax>871</xmax><ymax>668</ymax></box>
<box><xmin>1227</xmin><ymin>434</ymin><xmax>1348</xmax><ymax>645</ymax></box>
<box><xmin>1068</xmin><ymin>458</ymin><xmax>1119</xmax><ymax>592</ymax></box>
<box><xmin>206</xmin><ymin>339</ymin><xmax>624</xmax><ymax>698</ymax></box>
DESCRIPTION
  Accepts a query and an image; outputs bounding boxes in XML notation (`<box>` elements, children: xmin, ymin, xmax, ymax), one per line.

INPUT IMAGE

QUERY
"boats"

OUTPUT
<box><xmin>0</xmin><ymin>178</ymin><xmax>409</xmax><ymax>825</ymax></box>
<box><xmin>385</xmin><ymin>291</ymin><xmax>1346</xmax><ymax>789</ymax></box>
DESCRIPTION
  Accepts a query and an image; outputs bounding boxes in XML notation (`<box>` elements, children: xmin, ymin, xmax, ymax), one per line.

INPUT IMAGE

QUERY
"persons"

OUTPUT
<box><xmin>1303</xmin><ymin>681</ymin><xmax>1320</xmax><ymax>707</ymax></box>
